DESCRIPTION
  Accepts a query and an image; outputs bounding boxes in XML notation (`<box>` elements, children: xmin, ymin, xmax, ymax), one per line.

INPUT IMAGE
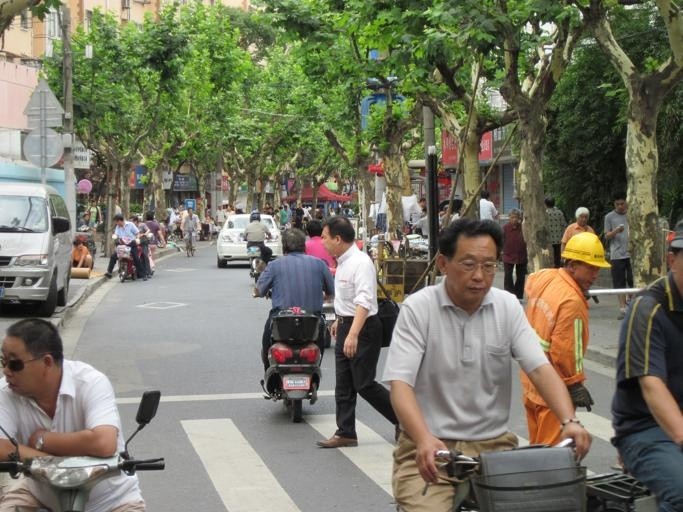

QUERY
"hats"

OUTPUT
<box><xmin>669</xmin><ymin>218</ymin><xmax>683</xmax><ymax>249</ymax></box>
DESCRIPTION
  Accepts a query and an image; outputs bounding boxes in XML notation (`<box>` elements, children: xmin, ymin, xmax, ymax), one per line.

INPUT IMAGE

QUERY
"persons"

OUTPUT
<box><xmin>544</xmin><ymin>197</ymin><xmax>567</xmax><ymax>270</ymax></box>
<box><xmin>74</xmin><ymin>198</ymin><xmax>201</xmax><ymax>282</ymax></box>
<box><xmin>607</xmin><ymin>224</ymin><xmax>682</xmax><ymax>512</ymax></box>
<box><xmin>603</xmin><ymin>191</ymin><xmax>635</xmax><ymax>321</ymax></box>
<box><xmin>204</xmin><ymin>204</ymin><xmax>237</xmax><ymax>227</ymax></box>
<box><xmin>244</xmin><ymin>211</ymin><xmax>274</xmax><ymax>265</ymax></box>
<box><xmin>316</xmin><ymin>214</ymin><xmax>401</xmax><ymax>449</ymax></box>
<box><xmin>251</xmin><ymin>226</ymin><xmax>335</xmax><ymax>372</ymax></box>
<box><xmin>501</xmin><ymin>208</ymin><xmax>528</xmax><ymax>301</ymax></box>
<box><xmin>561</xmin><ymin>205</ymin><xmax>594</xmax><ymax>263</ymax></box>
<box><xmin>0</xmin><ymin>317</ymin><xmax>150</xmax><ymax>512</ymax></box>
<box><xmin>403</xmin><ymin>189</ymin><xmax>499</xmax><ymax>238</ymax></box>
<box><xmin>520</xmin><ymin>230</ymin><xmax>612</xmax><ymax>449</ymax></box>
<box><xmin>265</xmin><ymin>201</ymin><xmax>353</xmax><ymax>232</ymax></box>
<box><xmin>380</xmin><ymin>215</ymin><xmax>594</xmax><ymax>512</ymax></box>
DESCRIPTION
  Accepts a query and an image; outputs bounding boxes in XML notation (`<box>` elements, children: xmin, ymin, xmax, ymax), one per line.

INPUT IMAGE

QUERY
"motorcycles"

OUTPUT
<box><xmin>110</xmin><ymin>233</ymin><xmax>149</xmax><ymax>282</ymax></box>
<box><xmin>0</xmin><ymin>389</ymin><xmax>164</xmax><ymax>511</ymax></box>
<box><xmin>252</xmin><ymin>286</ymin><xmax>325</xmax><ymax>425</ymax></box>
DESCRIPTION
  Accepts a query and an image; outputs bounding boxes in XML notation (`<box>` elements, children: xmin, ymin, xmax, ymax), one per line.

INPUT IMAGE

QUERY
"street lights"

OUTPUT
<box><xmin>85</xmin><ymin>41</ymin><xmax>95</xmax><ymax>147</ymax></box>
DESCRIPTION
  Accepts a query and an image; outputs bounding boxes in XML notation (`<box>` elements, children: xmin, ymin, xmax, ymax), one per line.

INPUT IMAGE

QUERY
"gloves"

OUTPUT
<box><xmin>568</xmin><ymin>382</ymin><xmax>594</xmax><ymax>412</ymax></box>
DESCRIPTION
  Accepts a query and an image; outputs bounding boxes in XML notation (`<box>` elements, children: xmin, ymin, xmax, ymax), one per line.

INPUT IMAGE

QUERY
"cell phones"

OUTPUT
<box><xmin>619</xmin><ymin>224</ymin><xmax>624</xmax><ymax>227</ymax></box>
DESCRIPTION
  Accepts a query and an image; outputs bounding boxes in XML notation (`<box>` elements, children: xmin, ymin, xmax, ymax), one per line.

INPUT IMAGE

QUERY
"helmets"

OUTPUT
<box><xmin>250</xmin><ymin>209</ymin><xmax>260</xmax><ymax>222</ymax></box>
<box><xmin>561</xmin><ymin>232</ymin><xmax>612</xmax><ymax>268</ymax></box>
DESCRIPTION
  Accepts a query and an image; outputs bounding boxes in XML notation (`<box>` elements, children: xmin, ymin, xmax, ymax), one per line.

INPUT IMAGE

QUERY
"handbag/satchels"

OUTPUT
<box><xmin>378</xmin><ymin>297</ymin><xmax>400</xmax><ymax>347</ymax></box>
<box><xmin>140</xmin><ymin>231</ymin><xmax>154</xmax><ymax>244</ymax></box>
<box><xmin>477</xmin><ymin>446</ymin><xmax>581</xmax><ymax>512</ymax></box>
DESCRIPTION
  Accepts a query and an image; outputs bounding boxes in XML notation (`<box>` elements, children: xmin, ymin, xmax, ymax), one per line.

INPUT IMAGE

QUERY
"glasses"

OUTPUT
<box><xmin>0</xmin><ymin>357</ymin><xmax>38</xmax><ymax>373</ymax></box>
<box><xmin>453</xmin><ymin>259</ymin><xmax>497</xmax><ymax>273</ymax></box>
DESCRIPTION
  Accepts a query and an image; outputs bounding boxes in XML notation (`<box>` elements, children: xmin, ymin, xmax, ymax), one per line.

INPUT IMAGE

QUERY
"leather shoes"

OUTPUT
<box><xmin>318</xmin><ymin>435</ymin><xmax>358</xmax><ymax>447</ymax></box>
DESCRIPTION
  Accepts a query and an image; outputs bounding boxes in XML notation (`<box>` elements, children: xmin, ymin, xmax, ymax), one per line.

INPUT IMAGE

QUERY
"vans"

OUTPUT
<box><xmin>0</xmin><ymin>180</ymin><xmax>74</xmax><ymax>320</ymax></box>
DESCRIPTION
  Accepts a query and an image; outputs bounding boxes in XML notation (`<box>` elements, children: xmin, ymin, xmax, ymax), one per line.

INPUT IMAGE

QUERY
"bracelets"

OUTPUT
<box><xmin>557</xmin><ymin>417</ymin><xmax>586</xmax><ymax>429</ymax></box>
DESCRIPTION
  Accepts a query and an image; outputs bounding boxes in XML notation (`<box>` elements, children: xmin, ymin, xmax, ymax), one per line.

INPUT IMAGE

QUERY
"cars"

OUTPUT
<box><xmin>217</xmin><ymin>213</ymin><xmax>282</xmax><ymax>269</ymax></box>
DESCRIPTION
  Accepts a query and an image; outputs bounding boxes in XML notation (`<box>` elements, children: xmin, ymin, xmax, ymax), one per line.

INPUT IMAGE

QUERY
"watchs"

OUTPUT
<box><xmin>32</xmin><ymin>429</ymin><xmax>45</xmax><ymax>450</ymax></box>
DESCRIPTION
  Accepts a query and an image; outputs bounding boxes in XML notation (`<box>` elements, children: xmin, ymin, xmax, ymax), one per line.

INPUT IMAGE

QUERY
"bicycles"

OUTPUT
<box><xmin>418</xmin><ymin>438</ymin><xmax>589</xmax><ymax>511</ymax></box>
<box><xmin>589</xmin><ymin>443</ymin><xmax>683</xmax><ymax>511</ymax></box>
<box><xmin>184</xmin><ymin>227</ymin><xmax>197</xmax><ymax>258</ymax></box>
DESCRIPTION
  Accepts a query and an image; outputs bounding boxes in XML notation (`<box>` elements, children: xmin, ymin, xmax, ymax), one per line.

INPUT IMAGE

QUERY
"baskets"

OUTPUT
<box><xmin>470</xmin><ymin>465</ymin><xmax>587</xmax><ymax>511</ymax></box>
<box><xmin>116</xmin><ymin>244</ymin><xmax>132</xmax><ymax>259</ymax></box>
